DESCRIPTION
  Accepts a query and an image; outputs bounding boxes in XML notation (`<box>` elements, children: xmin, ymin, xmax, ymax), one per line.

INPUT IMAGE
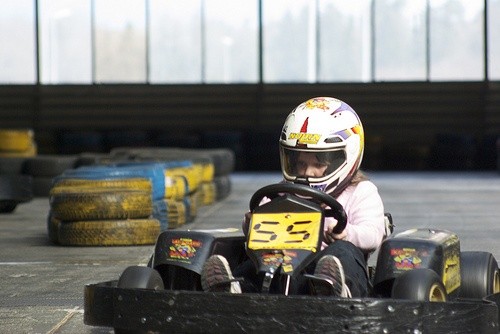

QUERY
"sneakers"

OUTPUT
<box><xmin>199</xmin><ymin>256</ymin><xmax>245</xmax><ymax>294</ymax></box>
<box><xmin>315</xmin><ymin>255</ymin><xmax>352</xmax><ymax>299</ymax></box>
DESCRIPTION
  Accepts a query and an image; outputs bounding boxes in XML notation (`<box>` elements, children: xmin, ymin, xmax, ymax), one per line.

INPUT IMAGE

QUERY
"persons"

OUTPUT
<box><xmin>200</xmin><ymin>96</ymin><xmax>385</xmax><ymax>300</ymax></box>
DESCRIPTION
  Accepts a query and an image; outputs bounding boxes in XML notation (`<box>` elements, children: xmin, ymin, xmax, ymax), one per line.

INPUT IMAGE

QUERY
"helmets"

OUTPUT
<box><xmin>279</xmin><ymin>96</ymin><xmax>365</xmax><ymax>203</ymax></box>
